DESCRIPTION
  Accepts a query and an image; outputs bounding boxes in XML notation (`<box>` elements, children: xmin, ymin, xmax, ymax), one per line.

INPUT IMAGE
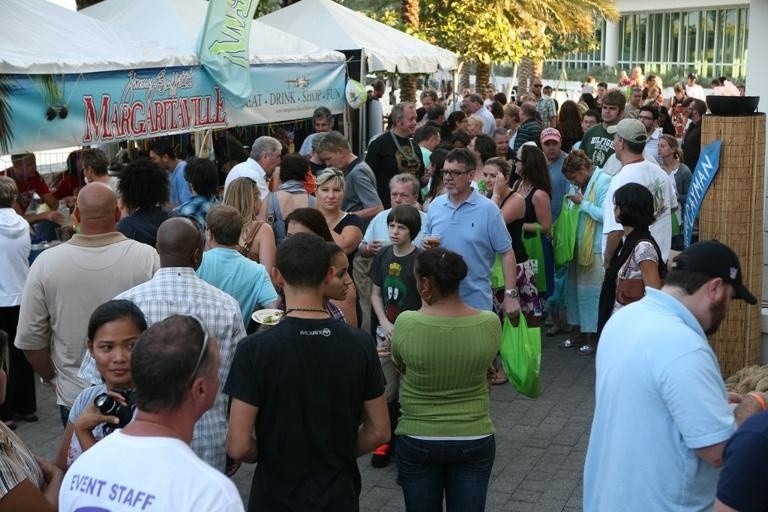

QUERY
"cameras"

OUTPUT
<box><xmin>95</xmin><ymin>390</ymin><xmax>131</xmax><ymax>427</ymax></box>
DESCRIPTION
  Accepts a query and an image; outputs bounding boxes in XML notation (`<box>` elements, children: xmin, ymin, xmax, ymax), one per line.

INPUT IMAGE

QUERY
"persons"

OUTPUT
<box><xmin>714</xmin><ymin>409</ymin><xmax>767</xmax><ymax>511</ymax></box>
<box><xmin>0</xmin><ymin>60</ymin><xmax>744</xmax><ymax>512</ymax></box>
<box><xmin>580</xmin><ymin>239</ymin><xmax>768</xmax><ymax>511</ymax></box>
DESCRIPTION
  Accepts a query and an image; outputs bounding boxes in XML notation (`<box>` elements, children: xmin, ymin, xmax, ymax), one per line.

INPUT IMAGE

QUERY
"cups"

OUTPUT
<box><xmin>374</xmin><ymin>238</ymin><xmax>392</xmax><ymax>255</ymax></box>
<box><xmin>425</xmin><ymin>232</ymin><xmax>441</xmax><ymax>248</ymax></box>
<box><xmin>377</xmin><ymin>325</ymin><xmax>387</xmax><ymax>347</ymax></box>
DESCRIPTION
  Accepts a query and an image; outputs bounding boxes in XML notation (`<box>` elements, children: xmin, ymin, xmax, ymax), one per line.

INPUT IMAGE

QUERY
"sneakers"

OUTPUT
<box><xmin>547</xmin><ymin>326</ymin><xmax>572</xmax><ymax>336</ymax></box>
<box><xmin>370</xmin><ymin>443</ymin><xmax>393</xmax><ymax>469</ymax></box>
<box><xmin>3</xmin><ymin>421</ymin><xmax>18</xmax><ymax>430</ymax></box>
<box><xmin>13</xmin><ymin>414</ymin><xmax>38</xmax><ymax>423</ymax></box>
<box><xmin>491</xmin><ymin>367</ymin><xmax>507</xmax><ymax>384</ymax></box>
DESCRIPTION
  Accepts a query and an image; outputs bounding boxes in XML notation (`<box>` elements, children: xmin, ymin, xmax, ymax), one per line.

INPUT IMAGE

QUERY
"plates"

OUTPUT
<box><xmin>251</xmin><ymin>308</ymin><xmax>286</xmax><ymax>326</ymax></box>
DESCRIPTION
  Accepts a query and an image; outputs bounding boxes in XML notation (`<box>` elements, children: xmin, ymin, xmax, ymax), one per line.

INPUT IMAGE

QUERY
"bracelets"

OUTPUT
<box><xmin>746</xmin><ymin>392</ymin><xmax>765</xmax><ymax>413</ymax></box>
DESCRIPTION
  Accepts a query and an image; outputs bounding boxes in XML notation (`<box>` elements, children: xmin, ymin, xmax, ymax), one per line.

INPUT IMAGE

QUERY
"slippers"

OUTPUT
<box><xmin>579</xmin><ymin>346</ymin><xmax>594</xmax><ymax>356</ymax></box>
<box><xmin>560</xmin><ymin>339</ymin><xmax>582</xmax><ymax>348</ymax></box>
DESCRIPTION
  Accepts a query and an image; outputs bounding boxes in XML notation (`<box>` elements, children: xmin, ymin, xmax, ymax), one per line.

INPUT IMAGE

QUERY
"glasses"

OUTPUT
<box><xmin>440</xmin><ymin>169</ymin><xmax>474</xmax><ymax>177</ymax></box>
<box><xmin>162</xmin><ymin>314</ymin><xmax>209</xmax><ymax>385</ymax></box>
<box><xmin>513</xmin><ymin>157</ymin><xmax>521</xmax><ymax>164</ymax></box>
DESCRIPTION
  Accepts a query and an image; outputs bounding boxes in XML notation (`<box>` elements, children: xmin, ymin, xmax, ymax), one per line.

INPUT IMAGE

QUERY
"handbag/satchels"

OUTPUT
<box><xmin>616</xmin><ymin>278</ymin><xmax>645</xmax><ymax>305</ymax></box>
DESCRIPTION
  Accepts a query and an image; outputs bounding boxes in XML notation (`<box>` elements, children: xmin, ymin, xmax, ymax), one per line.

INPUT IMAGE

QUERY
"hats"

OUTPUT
<box><xmin>671</xmin><ymin>239</ymin><xmax>757</xmax><ymax>304</ymax></box>
<box><xmin>607</xmin><ymin>119</ymin><xmax>647</xmax><ymax>143</ymax></box>
<box><xmin>540</xmin><ymin>127</ymin><xmax>562</xmax><ymax>143</ymax></box>
